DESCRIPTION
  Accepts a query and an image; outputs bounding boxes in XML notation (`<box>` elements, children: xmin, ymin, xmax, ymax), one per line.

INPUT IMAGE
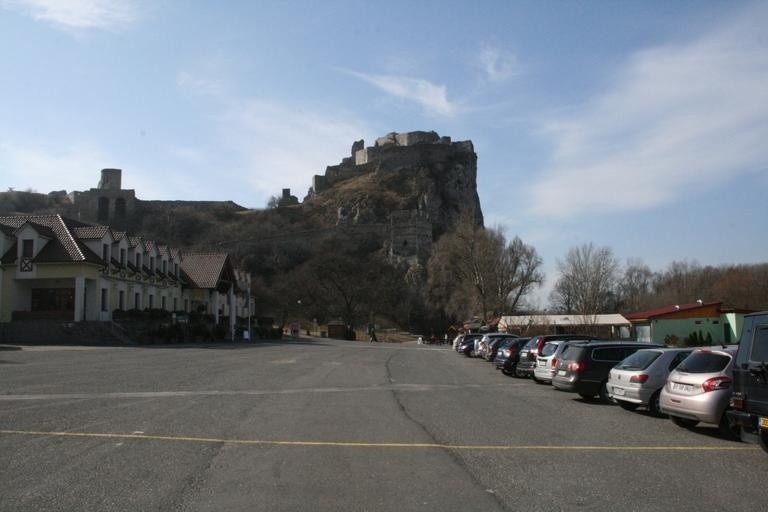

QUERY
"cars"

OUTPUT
<box><xmin>606</xmin><ymin>347</ymin><xmax>692</xmax><ymax>419</ymax></box>
<box><xmin>533</xmin><ymin>336</ymin><xmax>595</xmax><ymax>384</ymax></box>
<box><xmin>495</xmin><ymin>338</ymin><xmax>531</xmax><ymax>380</ymax></box>
<box><xmin>659</xmin><ymin>345</ymin><xmax>738</xmax><ymax>439</ymax></box>
<box><xmin>452</xmin><ymin>329</ymin><xmax>519</xmax><ymax>367</ymax></box>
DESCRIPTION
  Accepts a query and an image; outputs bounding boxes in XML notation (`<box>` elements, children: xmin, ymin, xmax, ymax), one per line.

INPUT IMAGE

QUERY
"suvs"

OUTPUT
<box><xmin>727</xmin><ymin>309</ymin><xmax>767</xmax><ymax>452</ymax></box>
<box><xmin>515</xmin><ymin>334</ymin><xmax>592</xmax><ymax>381</ymax></box>
<box><xmin>550</xmin><ymin>339</ymin><xmax>666</xmax><ymax>409</ymax></box>
